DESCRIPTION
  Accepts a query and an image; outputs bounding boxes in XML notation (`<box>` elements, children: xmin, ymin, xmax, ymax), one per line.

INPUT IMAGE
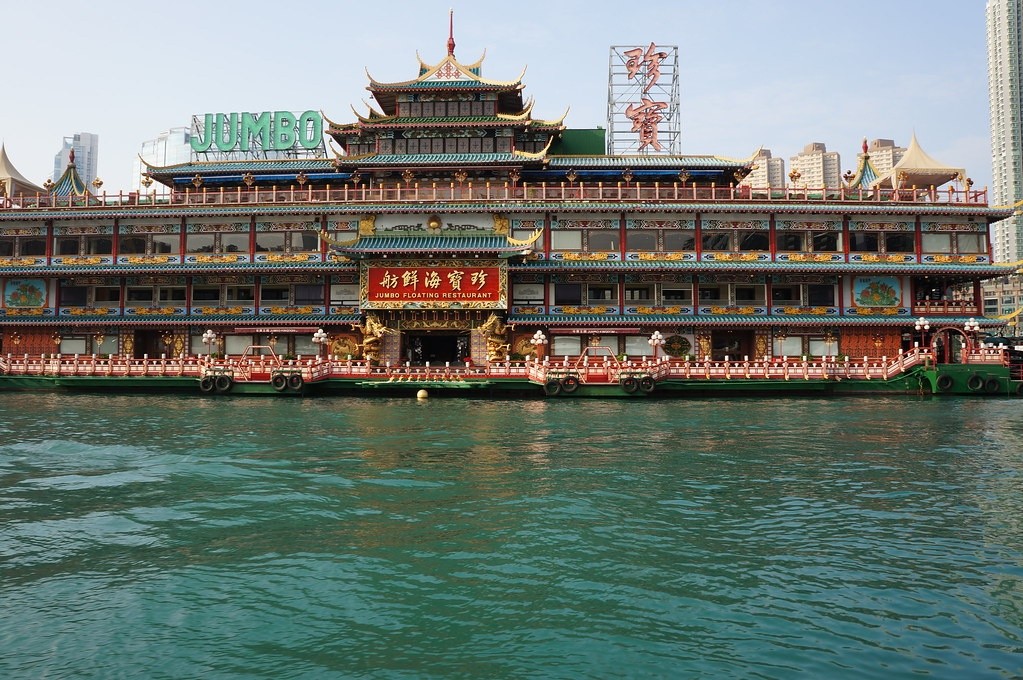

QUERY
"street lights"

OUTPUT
<box><xmin>312</xmin><ymin>328</ymin><xmax>328</xmax><ymax>356</ymax></box>
<box><xmin>202</xmin><ymin>329</ymin><xmax>217</xmax><ymax>355</ymax></box>
<box><xmin>648</xmin><ymin>330</ymin><xmax>666</xmax><ymax>359</ymax></box>
<box><xmin>964</xmin><ymin>317</ymin><xmax>980</xmax><ymax>349</ymax></box>
<box><xmin>915</xmin><ymin>316</ymin><xmax>930</xmax><ymax>347</ymax></box>
<box><xmin>530</xmin><ymin>329</ymin><xmax>548</xmax><ymax>358</ymax></box>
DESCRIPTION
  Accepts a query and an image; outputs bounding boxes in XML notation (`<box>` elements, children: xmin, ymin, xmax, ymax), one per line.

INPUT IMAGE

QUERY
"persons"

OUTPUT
<box><xmin>406</xmin><ymin>343</ymin><xmax>412</xmax><ymax>361</ymax></box>
<box><xmin>944</xmin><ymin>282</ymin><xmax>953</xmax><ymax>305</ymax></box>
